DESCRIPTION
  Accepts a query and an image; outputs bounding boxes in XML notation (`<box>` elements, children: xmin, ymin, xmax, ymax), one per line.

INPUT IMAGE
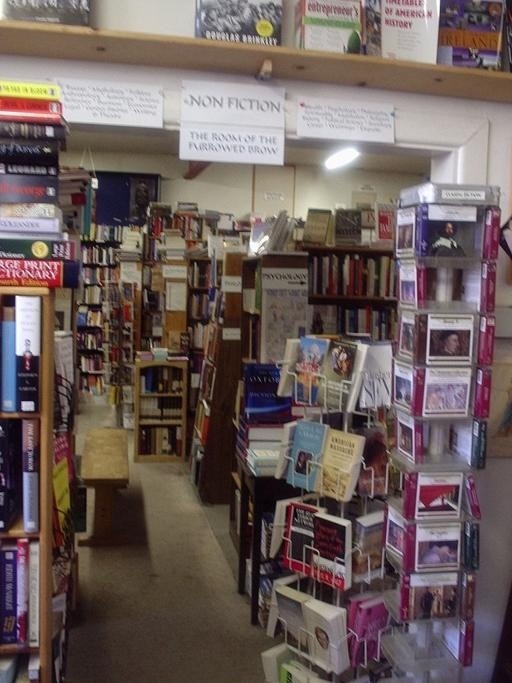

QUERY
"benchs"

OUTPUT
<box><xmin>76</xmin><ymin>429</ymin><xmax>133</xmax><ymax>547</ymax></box>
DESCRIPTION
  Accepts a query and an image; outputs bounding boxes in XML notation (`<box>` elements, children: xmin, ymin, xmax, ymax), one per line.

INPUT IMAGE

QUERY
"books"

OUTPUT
<box><xmin>196</xmin><ymin>1</ymin><xmax>512</xmax><ymax>72</ymax></box>
<box><xmin>0</xmin><ymin>80</ymin><xmax>499</xmax><ymax>683</ymax></box>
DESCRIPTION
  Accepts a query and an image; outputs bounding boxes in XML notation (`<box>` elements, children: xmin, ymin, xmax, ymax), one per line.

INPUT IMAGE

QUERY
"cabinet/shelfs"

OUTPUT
<box><xmin>83</xmin><ymin>221</ymin><xmax>190</xmax><ymax>462</ymax></box>
<box><xmin>187</xmin><ymin>247</ymin><xmax>498</xmax><ymax>683</ymax></box>
<box><xmin>0</xmin><ymin>285</ymin><xmax>78</xmax><ymax>683</ymax></box>
